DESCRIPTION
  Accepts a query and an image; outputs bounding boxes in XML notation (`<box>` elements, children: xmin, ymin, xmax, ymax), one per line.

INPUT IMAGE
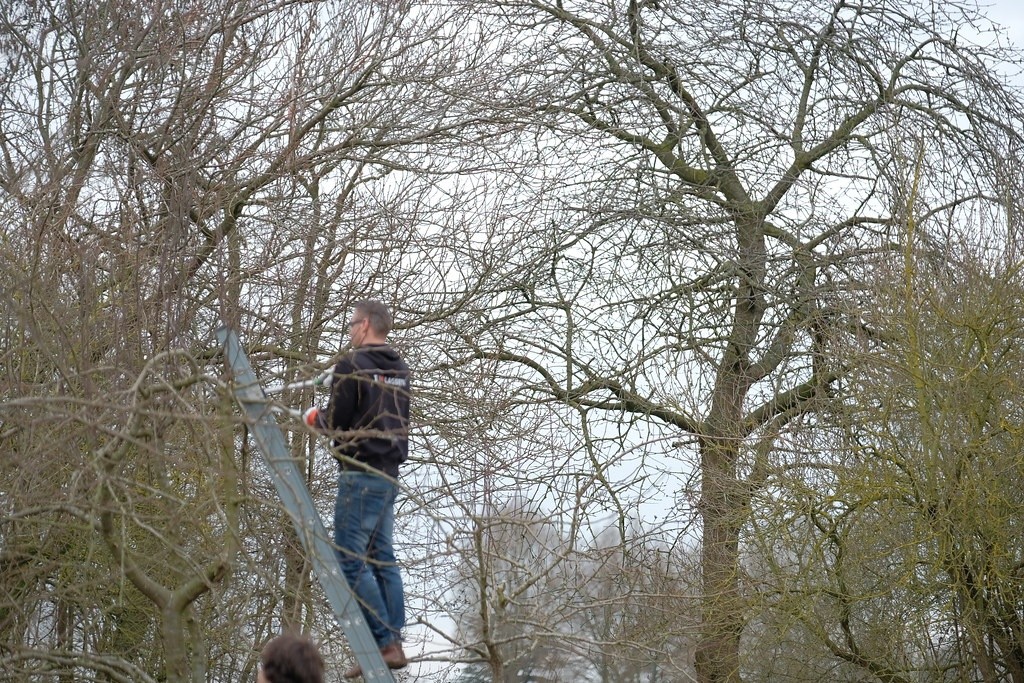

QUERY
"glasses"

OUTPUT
<box><xmin>348</xmin><ymin>320</ymin><xmax>363</xmax><ymax>327</ymax></box>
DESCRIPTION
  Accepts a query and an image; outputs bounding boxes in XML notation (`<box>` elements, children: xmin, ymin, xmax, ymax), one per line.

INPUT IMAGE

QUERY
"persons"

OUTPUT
<box><xmin>259</xmin><ymin>632</ymin><xmax>327</xmax><ymax>683</ymax></box>
<box><xmin>302</xmin><ymin>299</ymin><xmax>410</xmax><ymax>678</ymax></box>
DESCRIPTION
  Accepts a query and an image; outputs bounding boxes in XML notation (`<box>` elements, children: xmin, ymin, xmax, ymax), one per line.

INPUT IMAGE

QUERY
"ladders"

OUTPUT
<box><xmin>217</xmin><ymin>324</ymin><xmax>399</xmax><ymax>683</ymax></box>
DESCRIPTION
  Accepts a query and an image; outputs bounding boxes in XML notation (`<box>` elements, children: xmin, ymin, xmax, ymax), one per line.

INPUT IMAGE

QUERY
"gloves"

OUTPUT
<box><xmin>301</xmin><ymin>407</ymin><xmax>318</xmax><ymax>428</ymax></box>
